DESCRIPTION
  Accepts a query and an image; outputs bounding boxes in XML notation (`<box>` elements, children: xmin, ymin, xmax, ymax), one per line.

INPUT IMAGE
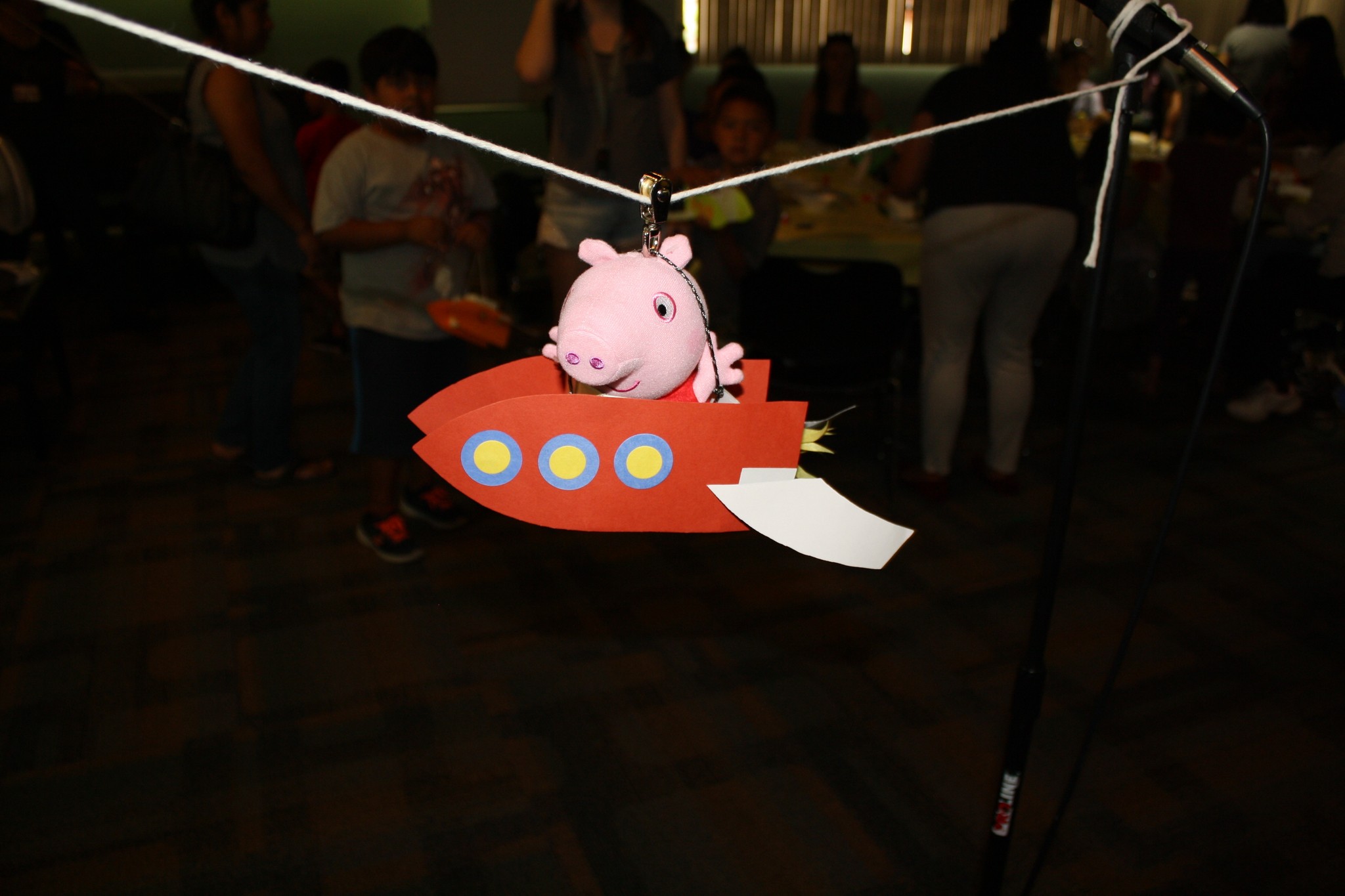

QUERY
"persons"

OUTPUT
<box><xmin>1</xmin><ymin>0</ymin><xmax>1345</xmax><ymax>563</ymax></box>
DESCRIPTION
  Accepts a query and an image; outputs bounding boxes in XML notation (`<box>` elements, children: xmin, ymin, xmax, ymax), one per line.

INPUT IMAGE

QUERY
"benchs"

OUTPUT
<box><xmin>683</xmin><ymin>55</ymin><xmax>968</xmax><ymax>143</ymax></box>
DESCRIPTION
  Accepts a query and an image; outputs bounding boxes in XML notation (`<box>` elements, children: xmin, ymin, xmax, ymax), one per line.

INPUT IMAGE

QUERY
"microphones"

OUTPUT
<box><xmin>1078</xmin><ymin>0</ymin><xmax>1268</xmax><ymax>121</ymax></box>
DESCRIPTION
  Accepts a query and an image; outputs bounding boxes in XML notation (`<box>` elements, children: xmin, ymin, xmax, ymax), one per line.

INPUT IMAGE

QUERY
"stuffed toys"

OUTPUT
<box><xmin>404</xmin><ymin>233</ymin><xmax>914</xmax><ymax>572</ymax></box>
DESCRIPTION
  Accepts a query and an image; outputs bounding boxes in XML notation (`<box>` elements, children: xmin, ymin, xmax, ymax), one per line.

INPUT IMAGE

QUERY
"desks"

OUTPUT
<box><xmin>733</xmin><ymin>179</ymin><xmax>920</xmax><ymax>464</ymax></box>
